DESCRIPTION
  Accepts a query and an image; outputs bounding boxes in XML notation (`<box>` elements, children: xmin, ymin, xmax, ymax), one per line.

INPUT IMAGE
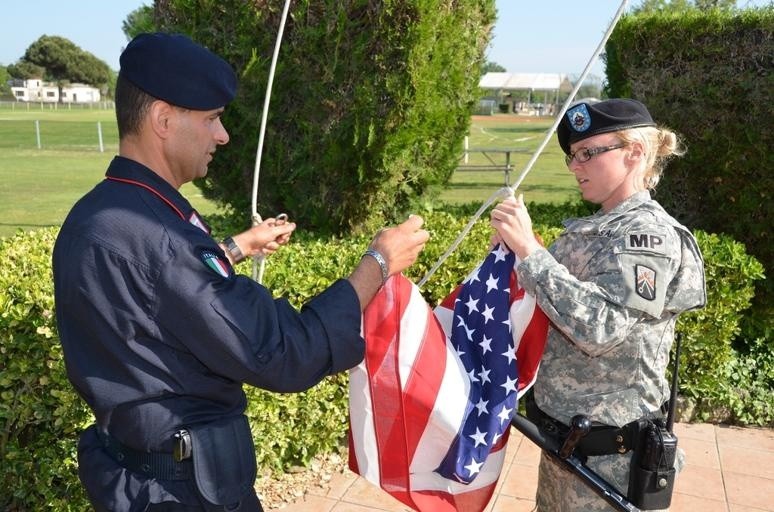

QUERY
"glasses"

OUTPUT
<box><xmin>564</xmin><ymin>144</ymin><xmax>629</xmax><ymax>165</ymax></box>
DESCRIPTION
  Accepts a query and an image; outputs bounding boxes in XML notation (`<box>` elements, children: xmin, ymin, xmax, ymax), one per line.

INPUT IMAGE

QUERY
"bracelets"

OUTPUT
<box><xmin>363</xmin><ymin>249</ymin><xmax>387</xmax><ymax>283</ymax></box>
<box><xmin>222</xmin><ymin>236</ymin><xmax>245</xmax><ymax>264</ymax></box>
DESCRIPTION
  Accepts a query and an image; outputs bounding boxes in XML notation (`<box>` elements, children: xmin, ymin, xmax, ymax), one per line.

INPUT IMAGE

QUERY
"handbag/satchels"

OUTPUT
<box><xmin>628</xmin><ymin>425</ymin><xmax>677</xmax><ymax>510</ymax></box>
<box><xmin>172</xmin><ymin>413</ymin><xmax>257</xmax><ymax>508</ymax></box>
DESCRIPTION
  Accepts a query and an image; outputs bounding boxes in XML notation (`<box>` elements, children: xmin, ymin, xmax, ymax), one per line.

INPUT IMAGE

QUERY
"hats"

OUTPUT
<box><xmin>557</xmin><ymin>97</ymin><xmax>657</xmax><ymax>156</ymax></box>
<box><xmin>120</xmin><ymin>33</ymin><xmax>236</xmax><ymax>110</ymax></box>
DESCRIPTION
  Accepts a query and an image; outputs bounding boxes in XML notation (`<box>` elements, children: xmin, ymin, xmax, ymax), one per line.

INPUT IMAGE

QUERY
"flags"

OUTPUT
<box><xmin>348</xmin><ymin>234</ymin><xmax>552</xmax><ymax>512</ymax></box>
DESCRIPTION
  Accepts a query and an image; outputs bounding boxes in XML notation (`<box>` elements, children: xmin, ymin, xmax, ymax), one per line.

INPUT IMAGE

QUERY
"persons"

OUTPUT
<box><xmin>53</xmin><ymin>33</ymin><xmax>430</xmax><ymax>512</ymax></box>
<box><xmin>488</xmin><ymin>97</ymin><xmax>708</xmax><ymax>512</ymax></box>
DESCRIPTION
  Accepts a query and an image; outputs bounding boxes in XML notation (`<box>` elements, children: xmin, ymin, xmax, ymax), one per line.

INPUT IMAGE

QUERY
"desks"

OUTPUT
<box><xmin>451</xmin><ymin>145</ymin><xmax>528</xmax><ymax>186</ymax></box>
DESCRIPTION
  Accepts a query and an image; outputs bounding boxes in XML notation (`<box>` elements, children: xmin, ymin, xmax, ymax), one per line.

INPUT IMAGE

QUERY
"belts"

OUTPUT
<box><xmin>526</xmin><ymin>398</ymin><xmax>641</xmax><ymax>454</ymax></box>
